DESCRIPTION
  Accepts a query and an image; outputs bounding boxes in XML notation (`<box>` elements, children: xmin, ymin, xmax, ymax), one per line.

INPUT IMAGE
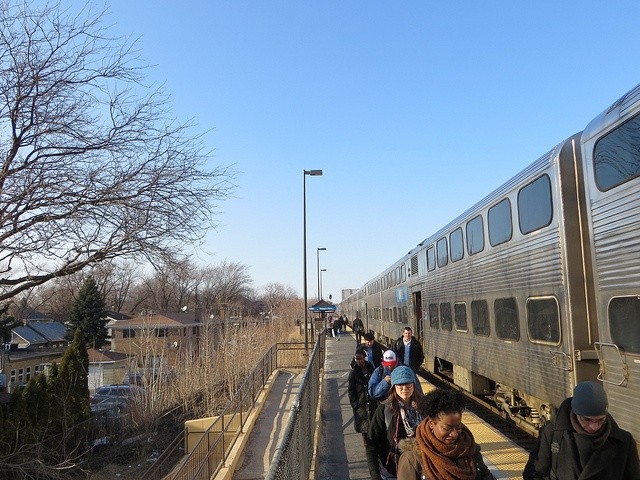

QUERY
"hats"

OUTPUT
<box><xmin>382</xmin><ymin>350</ymin><xmax>399</xmax><ymax>367</ymax></box>
<box><xmin>364</xmin><ymin>333</ymin><xmax>375</xmax><ymax>341</ymax></box>
<box><xmin>572</xmin><ymin>382</ymin><xmax>609</xmax><ymax>416</ymax></box>
<box><xmin>387</xmin><ymin>366</ymin><xmax>417</xmax><ymax>391</ymax></box>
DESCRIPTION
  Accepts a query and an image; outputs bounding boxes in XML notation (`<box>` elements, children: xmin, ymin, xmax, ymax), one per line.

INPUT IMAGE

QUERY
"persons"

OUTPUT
<box><xmin>353</xmin><ymin>315</ymin><xmax>364</xmax><ymax>345</ymax></box>
<box><xmin>393</xmin><ymin>327</ymin><xmax>424</xmax><ymax>374</ymax></box>
<box><xmin>348</xmin><ymin>349</ymin><xmax>377</xmax><ymax>446</ymax></box>
<box><xmin>356</xmin><ymin>333</ymin><xmax>382</xmax><ymax>369</ymax></box>
<box><xmin>522</xmin><ymin>381</ymin><xmax>640</xmax><ymax>480</ymax></box>
<box><xmin>397</xmin><ymin>386</ymin><xmax>494</xmax><ymax>480</ymax></box>
<box><xmin>368</xmin><ymin>350</ymin><xmax>423</xmax><ymax>407</ymax></box>
<box><xmin>332</xmin><ymin>314</ymin><xmax>348</xmax><ymax>341</ymax></box>
<box><xmin>366</xmin><ymin>366</ymin><xmax>424</xmax><ymax>480</ymax></box>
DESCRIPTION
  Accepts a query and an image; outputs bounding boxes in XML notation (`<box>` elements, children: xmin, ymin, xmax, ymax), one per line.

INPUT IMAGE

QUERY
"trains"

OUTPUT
<box><xmin>341</xmin><ymin>82</ymin><xmax>640</xmax><ymax>459</ymax></box>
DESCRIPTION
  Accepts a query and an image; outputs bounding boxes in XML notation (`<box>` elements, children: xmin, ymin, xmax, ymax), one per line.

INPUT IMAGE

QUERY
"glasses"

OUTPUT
<box><xmin>396</xmin><ymin>383</ymin><xmax>413</xmax><ymax>389</ymax></box>
<box><xmin>580</xmin><ymin>415</ymin><xmax>608</xmax><ymax>425</ymax></box>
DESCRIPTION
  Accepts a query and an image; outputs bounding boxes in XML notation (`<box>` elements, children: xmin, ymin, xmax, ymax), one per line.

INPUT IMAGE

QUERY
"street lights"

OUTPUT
<box><xmin>321</xmin><ymin>270</ymin><xmax>326</xmax><ymax>301</ymax></box>
<box><xmin>318</xmin><ymin>248</ymin><xmax>326</xmax><ymax>319</ymax></box>
<box><xmin>303</xmin><ymin>169</ymin><xmax>322</xmax><ymax>364</ymax></box>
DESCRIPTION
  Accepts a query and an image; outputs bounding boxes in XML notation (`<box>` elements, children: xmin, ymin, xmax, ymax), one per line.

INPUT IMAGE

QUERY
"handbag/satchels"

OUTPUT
<box><xmin>354</xmin><ymin>383</ymin><xmax>379</xmax><ymax>434</ymax></box>
<box><xmin>391</xmin><ymin>337</ymin><xmax>403</xmax><ymax>356</ymax></box>
